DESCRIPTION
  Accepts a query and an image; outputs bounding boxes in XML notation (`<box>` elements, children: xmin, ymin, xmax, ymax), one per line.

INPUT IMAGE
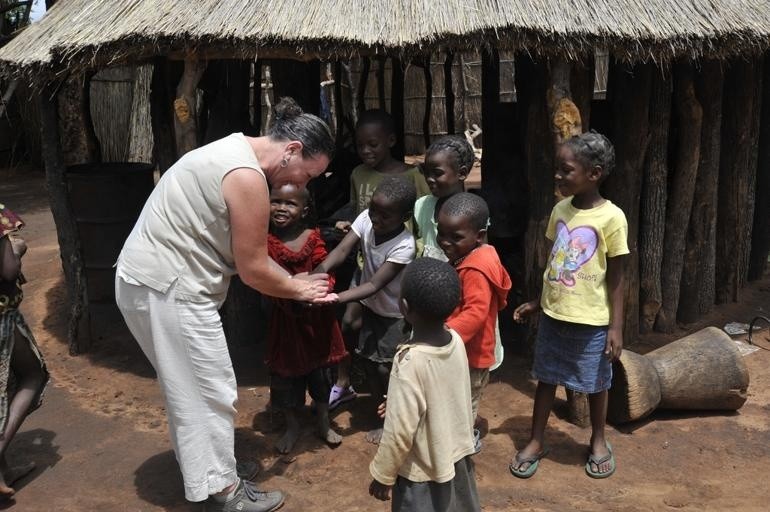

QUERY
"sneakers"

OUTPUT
<box><xmin>202</xmin><ymin>460</ymin><xmax>285</xmax><ymax>512</ymax></box>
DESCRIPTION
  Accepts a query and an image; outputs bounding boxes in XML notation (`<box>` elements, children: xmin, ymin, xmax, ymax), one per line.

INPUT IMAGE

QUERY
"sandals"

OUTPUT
<box><xmin>313</xmin><ymin>383</ymin><xmax>358</xmax><ymax>413</ymax></box>
<box><xmin>474</xmin><ymin>429</ymin><xmax>481</xmax><ymax>452</ymax></box>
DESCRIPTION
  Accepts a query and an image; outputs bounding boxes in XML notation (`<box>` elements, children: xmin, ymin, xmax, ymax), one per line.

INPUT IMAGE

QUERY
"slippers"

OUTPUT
<box><xmin>511</xmin><ymin>445</ymin><xmax>549</xmax><ymax>478</ymax></box>
<box><xmin>586</xmin><ymin>440</ymin><xmax>615</xmax><ymax>478</ymax></box>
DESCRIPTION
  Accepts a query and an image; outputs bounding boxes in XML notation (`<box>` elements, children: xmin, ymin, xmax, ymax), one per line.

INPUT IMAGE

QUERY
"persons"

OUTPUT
<box><xmin>508</xmin><ymin>128</ymin><xmax>630</xmax><ymax>479</ymax></box>
<box><xmin>111</xmin><ymin>95</ymin><xmax>332</xmax><ymax>511</ymax></box>
<box><xmin>0</xmin><ymin>201</ymin><xmax>50</xmax><ymax>499</ymax></box>
<box><xmin>269</xmin><ymin>107</ymin><xmax>511</xmax><ymax>511</ymax></box>
